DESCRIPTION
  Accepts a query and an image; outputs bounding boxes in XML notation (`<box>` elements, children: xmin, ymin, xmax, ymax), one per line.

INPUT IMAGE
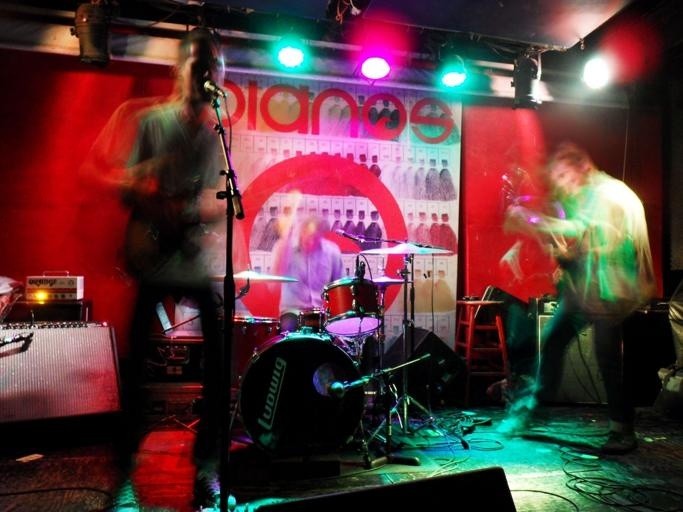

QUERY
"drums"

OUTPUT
<box><xmin>237</xmin><ymin>330</ymin><xmax>368</xmax><ymax>457</ymax></box>
<box><xmin>322</xmin><ymin>278</ymin><xmax>382</xmax><ymax>337</ymax></box>
<box><xmin>230</xmin><ymin>316</ymin><xmax>278</xmax><ymax>391</ymax></box>
<box><xmin>298</xmin><ymin>309</ymin><xmax>325</xmax><ymax>333</ymax></box>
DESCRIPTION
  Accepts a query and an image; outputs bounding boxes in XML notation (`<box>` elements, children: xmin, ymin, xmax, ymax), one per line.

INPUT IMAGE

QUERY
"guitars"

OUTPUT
<box><xmin>500</xmin><ymin>168</ymin><xmax>579</xmax><ymax>301</ymax></box>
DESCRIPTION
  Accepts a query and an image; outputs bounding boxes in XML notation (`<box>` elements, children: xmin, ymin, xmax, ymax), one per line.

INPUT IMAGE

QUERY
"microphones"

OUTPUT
<box><xmin>336</xmin><ymin>228</ymin><xmax>364</xmax><ymax>244</ymax></box>
<box><xmin>204</xmin><ymin>80</ymin><xmax>226</xmax><ymax>97</ymax></box>
<box><xmin>355</xmin><ymin>255</ymin><xmax>359</xmax><ymax>277</ymax></box>
<box><xmin>22</xmin><ymin>332</ymin><xmax>33</xmax><ymax>350</ymax></box>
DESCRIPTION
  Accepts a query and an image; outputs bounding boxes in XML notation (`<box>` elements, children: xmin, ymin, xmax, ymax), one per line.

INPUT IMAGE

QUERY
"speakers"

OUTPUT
<box><xmin>0</xmin><ymin>320</ymin><xmax>125</xmax><ymax>455</ymax></box>
<box><xmin>254</xmin><ymin>467</ymin><xmax>517</xmax><ymax>512</ymax></box>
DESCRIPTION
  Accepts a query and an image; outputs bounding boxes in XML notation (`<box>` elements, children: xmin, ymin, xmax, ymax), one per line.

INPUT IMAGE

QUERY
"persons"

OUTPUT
<box><xmin>273</xmin><ymin>203</ymin><xmax>343</xmax><ymax>334</ymax></box>
<box><xmin>78</xmin><ymin>29</ymin><xmax>230</xmax><ymax>512</ymax></box>
<box><xmin>498</xmin><ymin>144</ymin><xmax>656</xmax><ymax>454</ymax></box>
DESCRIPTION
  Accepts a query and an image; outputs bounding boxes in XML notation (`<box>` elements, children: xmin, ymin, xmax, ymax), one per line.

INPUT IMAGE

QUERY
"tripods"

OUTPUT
<box><xmin>345</xmin><ymin>353</ymin><xmax>432</xmax><ymax>468</ymax></box>
<box><xmin>355</xmin><ymin>258</ymin><xmax>469</xmax><ymax>456</ymax></box>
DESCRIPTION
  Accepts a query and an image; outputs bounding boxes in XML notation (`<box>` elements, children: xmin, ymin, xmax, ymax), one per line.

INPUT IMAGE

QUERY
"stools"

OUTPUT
<box><xmin>455</xmin><ymin>301</ymin><xmax>509</xmax><ymax>372</ymax></box>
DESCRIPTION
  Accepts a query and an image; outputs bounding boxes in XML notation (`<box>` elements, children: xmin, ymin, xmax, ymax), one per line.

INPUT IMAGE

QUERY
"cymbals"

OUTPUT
<box><xmin>361</xmin><ymin>245</ymin><xmax>453</xmax><ymax>255</ymax></box>
<box><xmin>374</xmin><ymin>277</ymin><xmax>408</xmax><ymax>283</ymax></box>
<box><xmin>213</xmin><ymin>271</ymin><xmax>301</xmax><ymax>282</ymax></box>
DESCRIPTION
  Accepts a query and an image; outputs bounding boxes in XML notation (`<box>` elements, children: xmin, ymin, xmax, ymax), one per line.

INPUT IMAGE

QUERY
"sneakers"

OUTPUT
<box><xmin>602</xmin><ymin>430</ymin><xmax>638</xmax><ymax>456</ymax></box>
<box><xmin>110</xmin><ymin>479</ymin><xmax>138</xmax><ymax>511</ymax></box>
<box><xmin>193</xmin><ymin>476</ymin><xmax>237</xmax><ymax>511</ymax></box>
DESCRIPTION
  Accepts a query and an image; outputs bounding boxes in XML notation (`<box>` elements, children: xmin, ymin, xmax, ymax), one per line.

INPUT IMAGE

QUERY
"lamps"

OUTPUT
<box><xmin>511</xmin><ymin>54</ymin><xmax>543</xmax><ymax>110</ymax></box>
